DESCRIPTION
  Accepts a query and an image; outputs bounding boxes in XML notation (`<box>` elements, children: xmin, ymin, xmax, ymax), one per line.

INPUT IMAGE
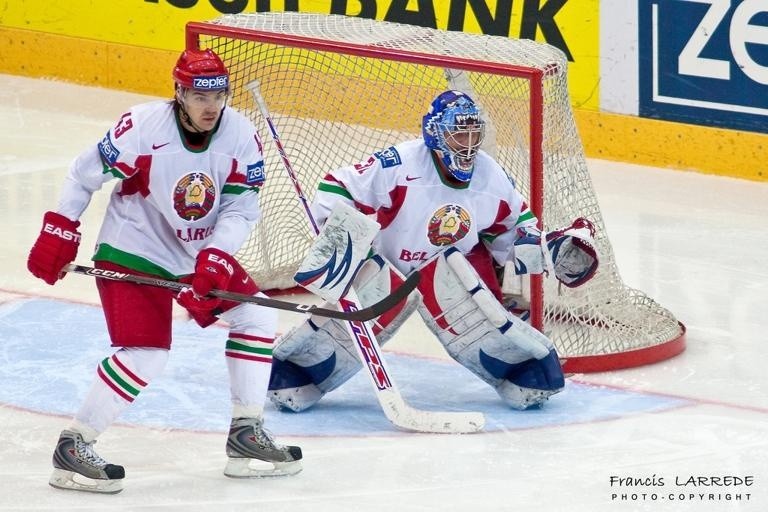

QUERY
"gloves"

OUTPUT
<box><xmin>177</xmin><ymin>248</ymin><xmax>234</xmax><ymax>310</ymax></box>
<box><xmin>29</xmin><ymin>212</ymin><xmax>81</xmax><ymax>285</ymax></box>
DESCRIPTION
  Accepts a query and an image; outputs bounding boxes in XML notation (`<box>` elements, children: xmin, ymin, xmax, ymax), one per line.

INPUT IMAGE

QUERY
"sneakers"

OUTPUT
<box><xmin>226</xmin><ymin>418</ymin><xmax>302</xmax><ymax>462</ymax></box>
<box><xmin>53</xmin><ymin>429</ymin><xmax>124</xmax><ymax>479</ymax></box>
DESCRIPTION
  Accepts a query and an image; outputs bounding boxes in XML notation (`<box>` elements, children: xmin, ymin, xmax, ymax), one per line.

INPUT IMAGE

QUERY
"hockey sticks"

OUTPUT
<box><xmin>63</xmin><ymin>263</ymin><xmax>420</xmax><ymax>321</ymax></box>
<box><xmin>245</xmin><ymin>79</ymin><xmax>486</xmax><ymax>434</ymax></box>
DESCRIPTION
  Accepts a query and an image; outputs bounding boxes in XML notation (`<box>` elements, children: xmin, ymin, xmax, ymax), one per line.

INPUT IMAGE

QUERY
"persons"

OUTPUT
<box><xmin>27</xmin><ymin>48</ymin><xmax>303</xmax><ymax>495</ymax></box>
<box><xmin>267</xmin><ymin>89</ymin><xmax>599</xmax><ymax>413</ymax></box>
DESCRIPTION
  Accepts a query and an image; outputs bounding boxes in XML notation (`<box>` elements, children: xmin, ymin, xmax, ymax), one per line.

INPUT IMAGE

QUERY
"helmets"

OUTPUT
<box><xmin>423</xmin><ymin>90</ymin><xmax>486</xmax><ymax>182</ymax></box>
<box><xmin>174</xmin><ymin>49</ymin><xmax>230</xmax><ymax>91</ymax></box>
